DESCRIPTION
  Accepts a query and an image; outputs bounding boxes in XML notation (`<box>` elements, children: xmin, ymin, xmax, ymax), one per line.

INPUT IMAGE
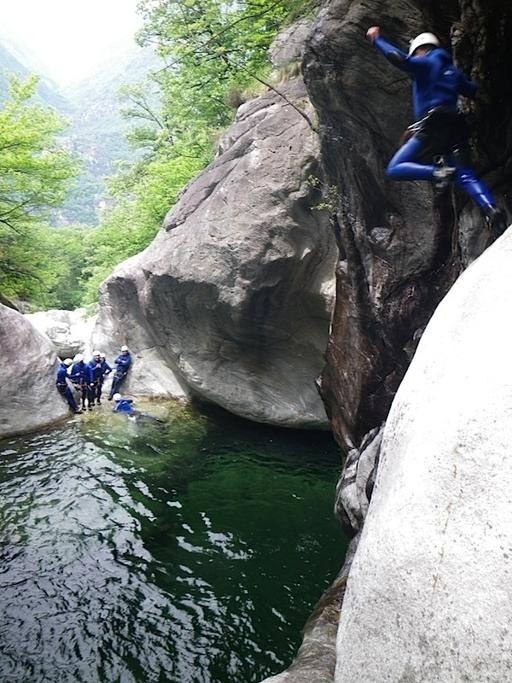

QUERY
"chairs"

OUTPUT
<box><xmin>75</xmin><ymin>400</ymin><xmax>101</xmax><ymax>413</ymax></box>
<box><xmin>432</xmin><ymin>167</ymin><xmax>457</xmax><ymax>210</ymax></box>
<box><xmin>483</xmin><ymin>207</ymin><xmax>508</xmax><ymax>242</ymax></box>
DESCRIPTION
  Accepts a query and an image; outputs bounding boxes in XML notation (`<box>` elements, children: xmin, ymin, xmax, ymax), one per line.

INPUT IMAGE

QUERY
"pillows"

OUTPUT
<box><xmin>63</xmin><ymin>351</ymin><xmax>105</xmax><ymax>366</ymax></box>
<box><xmin>113</xmin><ymin>393</ymin><xmax>121</xmax><ymax>402</ymax></box>
<box><xmin>121</xmin><ymin>345</ymin><xmax>129</xmax><ymax>352</ymax></box>
<box><xmin>408</xmin><ymin>32</ymin><xmax>439</xmax><ymax>57</ymax></box>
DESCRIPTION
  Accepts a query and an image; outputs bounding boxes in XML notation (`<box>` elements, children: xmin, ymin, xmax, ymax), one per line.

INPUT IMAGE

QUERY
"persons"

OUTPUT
<box><xmin>107</xmin><ymin>345</ymin><xmax>132</xmax><ymax>400</ymax></box>
<box><xmin>70</xmin><ymin>348</ymin><xmax>113</xmax><ymax>410</ymax></box>
<box><xmin>111</xmin><ymin>391</ymin><xmax>166</xmax><ymax>424</ymax></box>
<box><xmin>55</xmin><ymin>357</ymin><xmax>85</xmax><ymax>414</ymax></box>
<box><xmin>362</xmin><ymin>20</ymin><xmax>509</xmax><ymax>228</ymax></box>
